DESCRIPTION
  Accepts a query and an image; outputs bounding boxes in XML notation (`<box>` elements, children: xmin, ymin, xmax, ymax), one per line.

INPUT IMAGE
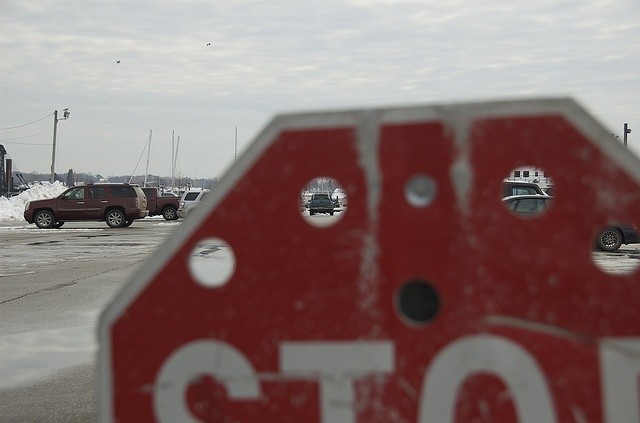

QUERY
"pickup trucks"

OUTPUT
<box><xmin>141</xmin><ymin>188</ymin><xmax>181</xmax><ymax>221</ymax></box>
<box><xmin>308</xmin><ymin>194</ymin><xmax>333</xmax><ymax>215</ymax></box>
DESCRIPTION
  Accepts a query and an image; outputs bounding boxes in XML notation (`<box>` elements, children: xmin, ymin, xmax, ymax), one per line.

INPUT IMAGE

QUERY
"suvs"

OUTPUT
<box><xmin>504</xmin><ymin>181</ymin><xmax>548</xmax><ymax>196</ymax></box>
<box><xmin>24</xmin><ymin>183</ymin><xmax>149</xmax><ymax>228</ymax></box>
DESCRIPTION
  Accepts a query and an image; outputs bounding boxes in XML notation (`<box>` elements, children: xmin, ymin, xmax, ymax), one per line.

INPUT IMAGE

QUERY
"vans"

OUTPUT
<box><xmin>177</xmin><ymin>190</ymin><xmax>210</xmax><ymax>218</ymax></box>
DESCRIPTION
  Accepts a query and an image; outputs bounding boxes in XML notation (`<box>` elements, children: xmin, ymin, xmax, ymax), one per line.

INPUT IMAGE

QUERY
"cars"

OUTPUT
<box><xmin>162</xmin><ymin>192</ymin><xmax>177</xmax><ymax>197</ymax></box>
<box><xmin>502</xmin><ymin>195</ymin><xmax>640</xmax><ymax>251</ymax></box>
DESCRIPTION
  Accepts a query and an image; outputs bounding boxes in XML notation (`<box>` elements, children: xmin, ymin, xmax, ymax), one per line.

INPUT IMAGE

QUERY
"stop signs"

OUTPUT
<box><xmin>100</xmin><ymin>97</ymin><xmax>640</xmax><ymax>422</ymax></box>
